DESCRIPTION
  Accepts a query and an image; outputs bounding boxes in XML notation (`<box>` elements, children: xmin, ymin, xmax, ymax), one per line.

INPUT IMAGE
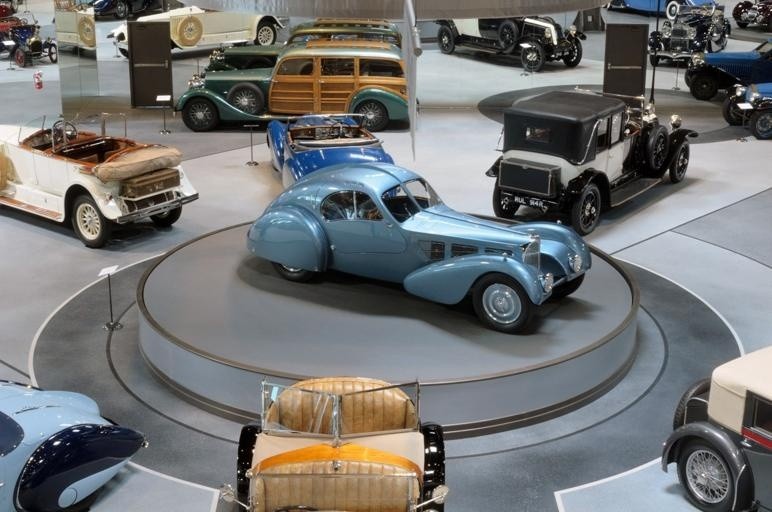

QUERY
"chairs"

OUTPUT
<box><xmin>248</xmin><ymin>377</ymin><xmax>423</xmax><ymax>511</ymax></box>
<box><xmin>56</xmin><ymin>136</ymin><xmax>145</xmax><ymax>163</ymax></box>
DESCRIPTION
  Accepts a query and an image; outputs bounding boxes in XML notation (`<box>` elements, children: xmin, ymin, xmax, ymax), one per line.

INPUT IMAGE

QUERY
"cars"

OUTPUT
<box><xmin>265</xmin><ymin>114</ymin><xmax>393</xmax><ymax>189</ymax></box>
<box><xmin>661</xmin><ymin>346</ymin><xmax>770</xmax><ymax>512</ymax></box>
<box><xmin>86</xmin><ymin>0</ymin><xmax>164</xmax><ymax>19</ymax></box>
<box><xmin>211</xmin><ymin>371</ymin><xmax>448</xmax><ymax>512</ymax></box>
<box><xmin>106</xmin><ymin>5</ymin><xmax>291</xmax><ymax>60</ymax></box>
<box><xmin>173</xmin><ymin>17</ymin><xmax>411</xmax><ymax>135</ymax></box>
<box><xmin>438</xmin><ymin>14</ymin><xmax>587</xmax><ymax>71</ymax></box>
<box><xmin>649</xmin><ymin>0</ymin><xmax>772</xmax><ymax>139</ymax></box>
<box><xmin>605</xmin><ymin>0</ymin><xmax>725</xmax><ymax>20</ymax></box>
<box><xmin>0</xmin><ymin>109</ymin><xmax>200</xmax><ymax>248</ymax></box>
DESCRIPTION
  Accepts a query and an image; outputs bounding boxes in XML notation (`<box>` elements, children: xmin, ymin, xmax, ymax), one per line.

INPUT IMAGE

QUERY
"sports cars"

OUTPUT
<box><xmin>243</xmin><ymin>159</ymin><xmax>592</xmax><ymax>334</ymax></box>
<box><xmin>0</xmin><ymin>359</ymin><xmax>149</xmax><ymax>507</ymax></box>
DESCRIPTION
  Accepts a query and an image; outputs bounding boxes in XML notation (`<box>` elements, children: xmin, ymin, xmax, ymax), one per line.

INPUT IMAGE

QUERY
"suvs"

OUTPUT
<box><xmin>487</xmin><ymin>86</ymin><xmax>699</xmax><ymax>237</ymax></box>
